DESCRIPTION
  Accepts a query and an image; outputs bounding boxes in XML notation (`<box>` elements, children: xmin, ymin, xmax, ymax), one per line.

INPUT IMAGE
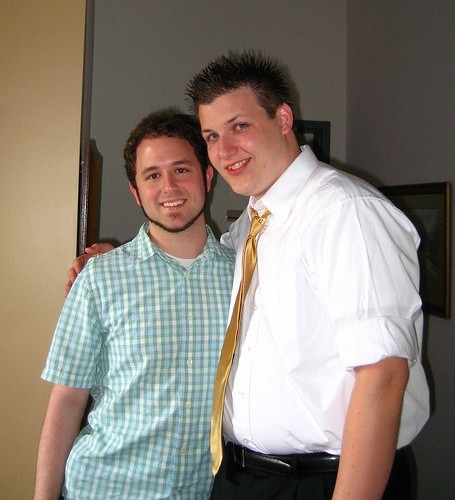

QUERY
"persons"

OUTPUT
<box><xmin>34</xmin><ymin>115</ymin><xmax>236</xmax><ymax>500</ymax></box>
<box><xmin>64</xmin><ymin>47</ymin><xmax>429</xmax><ymax>500</ymax></box>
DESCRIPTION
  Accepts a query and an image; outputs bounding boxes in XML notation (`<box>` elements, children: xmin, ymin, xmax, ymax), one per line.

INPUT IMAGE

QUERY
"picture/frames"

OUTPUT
<box><xmin>376</xmin><ymin>181</ymin><xmax>453</xmax><ymax>321</ymax></box>
<box><xmin>80</xmin><ymin>137</ymin><xmax>105</xmax><ymax>255</ymax></box>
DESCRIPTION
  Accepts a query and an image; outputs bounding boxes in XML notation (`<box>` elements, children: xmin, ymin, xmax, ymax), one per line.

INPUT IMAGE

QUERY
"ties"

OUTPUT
<box><xmin>209</xmin><ymin>208</ymin><xmax>271</xmax><ymax>475</ymax></box>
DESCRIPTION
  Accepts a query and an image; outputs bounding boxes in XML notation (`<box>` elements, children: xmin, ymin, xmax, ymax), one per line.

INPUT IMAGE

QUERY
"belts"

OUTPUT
<box><xmin>222</xmin><ymin>445</ymin><xmax>339</xmax><ymax>473</ymax></box>
